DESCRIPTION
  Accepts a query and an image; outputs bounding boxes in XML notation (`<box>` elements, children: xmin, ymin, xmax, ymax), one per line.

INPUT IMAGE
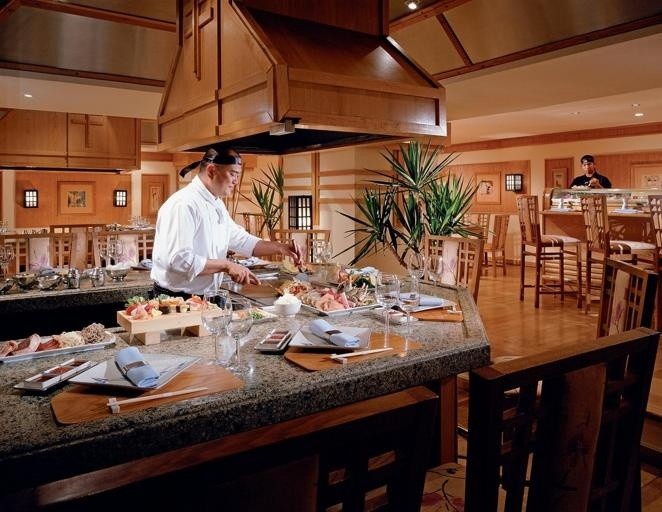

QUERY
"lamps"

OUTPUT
<box><xmin>504</xmin><ymin>173</ymin><xmax>525</xmax><ymax>193</ymax></box>
<box><xmin>112</xmin><ymin>187</ymin><xmax>129</xmax><ymax>208</ymax></box>
<box><xmin>19</xmin><ymin>186</ymin><xmax>39</xmax><ymax>210</ymax></box>
<box><xmin>288</xmin><ymin>194</ymin><xmax>314</xmax><ymax>264</ymax></box>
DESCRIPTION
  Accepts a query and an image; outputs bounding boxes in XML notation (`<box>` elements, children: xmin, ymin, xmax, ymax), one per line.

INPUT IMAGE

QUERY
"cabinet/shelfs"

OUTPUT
<box><xmin>0</xmin><ymin>107</ymin><xmax>143</xmax><ymax>174</ymax></box>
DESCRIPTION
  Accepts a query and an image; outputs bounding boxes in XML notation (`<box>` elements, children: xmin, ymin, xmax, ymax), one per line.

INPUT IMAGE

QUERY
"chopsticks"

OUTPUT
<box><xmin>105</xmin><ymin>385</ymin><xmax>210</xmax><ymax>406</ymax></box>
<box><xmin>329</xmin><ymin>344</ymin><xmax>396</xmax><ymax>363</ymax></box>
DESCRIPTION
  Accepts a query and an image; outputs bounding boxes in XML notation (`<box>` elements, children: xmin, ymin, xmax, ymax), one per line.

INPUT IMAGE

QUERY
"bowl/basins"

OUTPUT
<box><xmin>274</xmin><ymin>300</ymin><xmax>303</xmax><ymax>318</ymax></box>
<box><xmin>10</xmin><ymin>272</ymin><xmax>63</xmax><ymax>291</ymax></box>
<box><xmin>105</xmin><ymin>268</ymin><xmax>131</xmax><ymax>280</ymax></box>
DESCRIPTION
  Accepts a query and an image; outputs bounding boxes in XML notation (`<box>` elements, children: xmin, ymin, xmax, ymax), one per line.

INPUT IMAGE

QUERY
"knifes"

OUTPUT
<box><xmin>246</xmin><ymin>275</ymin><xmax>286</xmax><ymax>298</ymax></box>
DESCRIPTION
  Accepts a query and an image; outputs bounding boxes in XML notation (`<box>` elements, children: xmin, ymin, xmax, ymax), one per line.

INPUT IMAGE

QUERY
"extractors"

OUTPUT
<box><xmin>152</xmin><ymin>1</ymin><xmax>449</xmax><ymax>157</ymax></box>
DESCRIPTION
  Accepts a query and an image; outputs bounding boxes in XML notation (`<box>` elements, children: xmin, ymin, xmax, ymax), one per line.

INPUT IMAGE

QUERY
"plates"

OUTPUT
<box><xmin>296</xmin><ymin>299</ymin><xmax>379</xmax><ymax>316</ymax></box>
<box><xmin>0</xmin><ymin>325</ymin><xmax>115</xmax><ymax>366</ymax></box>
<box><xmin>286</xmin><ymin>324</ymin><xmax>374</xmax><ymax>350</ymax></box>
<box><xmin>254</xmin><ymin>326</ymin><xmax>293</xmax><ymax>353</ymax></box>
<box><xmin>67</xmin><ymin>352</ymin><xmax>202</xmax><ymax>394</ymax></box>
<box><xmin>395</xmin><ymin>290</ymin><xmax>455</xmax><ymax>312</ymax></box>
<box><xmin>236</xmin><ymin>257</ymin><xmax>270</xmax><ymax>269</ymax></box>
<box><xmin>13</xmin><ymin>359</ymin><xmax>95</xmax><ymax>392</ymax></box>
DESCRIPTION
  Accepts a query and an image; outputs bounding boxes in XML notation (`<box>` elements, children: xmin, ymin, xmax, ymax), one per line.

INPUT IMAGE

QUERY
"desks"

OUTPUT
<box><xmin>536</xmin><ymin>206</ymin><xmax>653</xmax><ymax>309</ymax></box>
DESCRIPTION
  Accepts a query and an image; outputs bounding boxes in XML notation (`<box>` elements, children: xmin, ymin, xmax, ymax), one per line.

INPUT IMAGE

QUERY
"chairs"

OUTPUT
<box><xmin>512</xmin><ymin>195</ymin><xmax>583</xmax><ymax>310</ymax></box>
<box><xmin>579</xmin><ymin>194</ymin><xmax>654</xmax><ymax>311</ymax></box>
<box><xmin>270</xmin><ymin>228</ymin><xmax>331</xmax><ymax>265</ymax></box>
<box><xmin>0</xmin><ymin>385</ymin><xmax>440</xmax><ymax>511</ymax></box>
<box><xmin>647</xmin><ymin>193</ymin><xmax>662</xmax><ymax>252</ymax></box>
<box><xmin>0</xmin><ymin>232</ymin><xmax>79</xmax><ymax>277</ymax></box>
<box><xmin>484</xmin><ymin>212</ymin><xmax>511</xmax><ymax>278</ymax></box>
<box><xmin>50</xmin><ymin>222</ymin><xmax>107</xmax><ymax>272</ymax></box>
<box><xmin>89</xmin><ymin>228</ymin><xmax>157</xmax><ymax>269</ymax></box>
<box><xmin>593</xmin><ymin>255</ymin><xmax>657</xmax><ymax>337</ymax></box>
<box><xmin>418</xmin><ymin>327</ymin><xmax>660</xmax><ymax>512</ymax></box>
<box><xmin>418</xmin><ymin>234</ymin><xmax>486</xmax><ymax>305</ymax></box>
<box><xmin>476</xmin><ymin>213</ymin><xmax>491</xmax><ymax>242</ymax></box>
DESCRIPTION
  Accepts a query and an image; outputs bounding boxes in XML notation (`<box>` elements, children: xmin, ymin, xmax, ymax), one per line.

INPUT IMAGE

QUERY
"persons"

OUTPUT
<box><xmin>569</xmin><ymin>155</ymin><xmax>612</xmax><ymax>188</ymax></box>
<box><xmin>149</xmin><ymin>143</ymin><xmax>305</xmax><ymax>307</ymax></box>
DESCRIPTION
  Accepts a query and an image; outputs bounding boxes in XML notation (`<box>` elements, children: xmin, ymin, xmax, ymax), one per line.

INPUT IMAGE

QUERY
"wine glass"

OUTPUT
<box><xmin>424</xmin><ymin>254</ymin><xmax>447</xmax><ymax>298</ymax></box>
<box><xmin>97</xmin><ymin>239</ymin><xmax>124</xmax><ymax>266</ymax></box>
<box><xmin>395</xmin><ymin>274</ymin><xmax>421</xmax><ymax>342</ymax></box>
<box><xmin>373</xmin><ymin>270</ymin><xmax>401</xmax><ymax>332</ymax></box>
<box><xmin>201</xmin><ymin>288</ymin><xmax>232</xmax><ymax>368</ymax></box>
<box><xmin>223</xmin><ymin>297</ymin><xmax>254</xmax><ymax>376</ymax></box>
<box><xmin>314</xmin><ymin>238</ymin><xmax>333</xmax><ymax>281</ymax></box>
<box><xmin>406</xmin><ymin>251</ymin><xmax>425</xmax><ymax>279</ymax></box>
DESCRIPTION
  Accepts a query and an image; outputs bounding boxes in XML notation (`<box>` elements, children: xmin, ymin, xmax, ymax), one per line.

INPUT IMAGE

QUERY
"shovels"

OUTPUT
<box><xmin>250</xmin><ymin>279</ymin><xmax>284</xmax><ymax>297</ymax></box>
<box><xmin>292</xmin><ymin>239</ymin><xmax>309</xmax><ymax>284</ymax></box>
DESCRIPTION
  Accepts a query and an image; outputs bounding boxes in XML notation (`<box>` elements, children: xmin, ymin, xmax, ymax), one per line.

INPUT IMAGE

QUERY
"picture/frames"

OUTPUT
<box><xmin>56</xmin><ymin>179</ymin><xmax>97</xmax><ymax>216</ymax></box>
<box><xmin>147</xmin><ymin>182</ymin><xmax>165</xmax><ymax>219</ymax></box>
<box><xmin>627</xmin><ymin>162</ymin><xmax>662</xmax><ymax>191</ymax></box>
<box><xmin>472</xmin><ymin>170</ymin><xmax>501</xmax><ymax>206</ymax></box>
<box><xmin>550</xmin><ymin>167</ymin><xmax>567</xmax><ymax>190</ymax></box>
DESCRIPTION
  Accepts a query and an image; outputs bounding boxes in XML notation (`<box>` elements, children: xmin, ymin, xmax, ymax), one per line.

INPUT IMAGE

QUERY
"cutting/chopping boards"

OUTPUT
<box><xmin>51</xmin><ymin>366</ymin><xmax>244</xmax><ymax>427</ymax></box>
<box><xmin>283</xmin><ymin>333</ymin><xmax>423</xmax><ymax>373</ymax></box>
<box><xmin>405</xmin><ymin>306</ymin><xmax>464</xmax><ymax>324</ymax></box>
